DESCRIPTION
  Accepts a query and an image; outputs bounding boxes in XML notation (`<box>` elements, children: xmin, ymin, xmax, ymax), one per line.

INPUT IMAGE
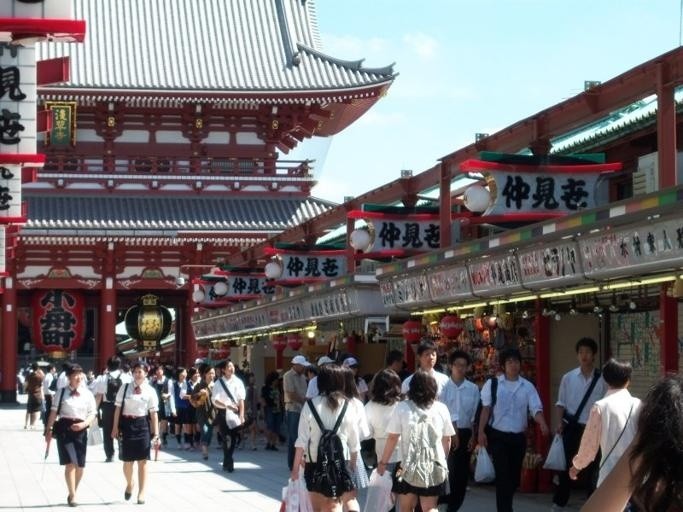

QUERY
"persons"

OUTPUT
<box><xmin>292</xmin><ymin>363</ymin><xmax>360</xmax><ymax>511</ymax></box>
<box><xmin>180</xmin><ymin>367</ymin><xmax>202</xmax><ymax>448</ymax></box>
<box><xmin>478</xmin><ymin>347</ymin><xmax>548</xmax><ymax>510</ymax></box>
<box><xmin>43</xmin><ymin>365</ymin><xmax>57</xmax><ymax>425</ymax></box>
<box><xmin>149</xmin><ymin>365</ymin><xmax>173</xmax><ymax>448</ymax></box>
<box><xmin>399</xmin><ymin>340</ymin><xmax>459</xmax><ymax>450</ymax></box>
<box><xmin>345</xmin><ymin>358</ymin><xmax>368</xmax><ymax>400</ymax></box>
<box><xmin>172</xmin><ymin>366</ymin><xmax>191</xmax><ymax>449</ymax></box>
<box><xmin>238</xmin><ymin>371</ymin><xmax>258</xmax><ymax>450</ymax></box>
<box><xmin>378</xmin><ymin>373</ymin><xmax>454</xmax><ymax>512</ymax></box>
<box><xmin>573</xmin><ymin>372</ymin><xmax>683</xmax><ymax>512</ymax></box>
<box><xmin>263</xmin><ymin>371</ymin><xmax>283</xmax><ymax>450</ymax></box>
<box><xmin>109</xmin><ymin>363</ymin><xmax>160</xmax><ymax>505</ymax></box>
<box><xmin>214</xmin><ymin>359</ymin><xmax>246</xmax><ymax>472</ymax></box>
<box><xmin>283</xmin><ymin>355</ymin><xmax>310</xmax><ymax>470</ymax></box>
<box><xmin>190</xmin><ymin>366</ymin><xmax>217</xmax><ymax>462</ymax></box>
<box><xmin>364</xmin><ymin>370</ymin><xmax>405</xmax><ymax>474</ymax></box>
<box><xmin>23</xmin><ymin>363</ymin><xmax>43</xmax><ymax>428</ymax></box>
<box><xmin>120</xmin><ymin>364</ymin><xmax>133</xmax><ymax>382</ymax></box>
<box><xmin>305</xmin><ymin>356</ymin><xmax>335</xmax><ymax>402</ymax></box>
<box><xmin>45</xmin><ymin>363</ymin><xmax>97</xmax><ymax>506</ymax></box>
<box><xmin>94</xmin><ymin>355</ymin><xmax>132</xmax><ymax>462</ymax></box>
<box><xmin>570</xmin><ymin>356</ymin><xmax>641</xmax><ymax>490</ymax></box>
<box><xmin>377</xmin><ymin>349</ymin><xmax>404</xmax><ymax>375</ymax></box>
<box><xmin>449</xmin><ymin>350</ymin><xmax>479</xmax><ymax>511</ymax></box>
<box><xmin>552</xmin><ymin>339</ymin><xmax>607</xmax><ymax>509</ymax></box>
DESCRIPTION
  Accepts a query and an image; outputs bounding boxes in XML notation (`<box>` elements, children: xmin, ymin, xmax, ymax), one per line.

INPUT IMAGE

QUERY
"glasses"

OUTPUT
<box><xmin>449</xmin><ymin>360</ymin><xmax>465</xmax><ymax>367</ymax></box>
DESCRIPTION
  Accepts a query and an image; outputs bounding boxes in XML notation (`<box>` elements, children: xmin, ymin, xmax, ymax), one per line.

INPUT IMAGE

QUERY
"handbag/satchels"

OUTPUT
<box><xmin>561</xmin><ymin>368</ymin><xmax>599</xmax><ymax>455</ymax></box>
<box><xmin>217</xmin><ymin>376</ymin><xmax>251</xmax><ymax>432</ymax></box>
<box><xmin>473</xmin><ymin>374</ymin><xmax>499</xmax><ymax>426</ymax></box>
<box><xmin>44</xmin><ymin>387</ymin><xmax>65</xmax><ymax>439</ymax></box>
<box><xmin>190</xmin><ymin>381</ymin><xmax>211</xmax><ymax>408</ymax></box>
<box><xmin>115</xmin><ymin>383</ymin><xmax>127</xmax><ymax>438</ymax></box>
<box><xmin>580</xmin><ymin>401</ymin><xmax>633</xmax><ymax>482</ymax></box>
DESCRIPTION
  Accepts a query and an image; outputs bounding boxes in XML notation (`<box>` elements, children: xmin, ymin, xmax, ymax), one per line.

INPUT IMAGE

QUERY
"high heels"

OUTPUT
<box><xmin>67</xmin><ymin>494</ymin><xmax>78</xmax><ymax>508</ymax></box>
<box><xmin>124</xmin><ymin>488</ymin><xmax>131</xmax><ymax>499</ymax></box>
<box><xmin>138</xmin><ymin>497</ymin><xmax>142</xmax><ymax>503</ymax></box>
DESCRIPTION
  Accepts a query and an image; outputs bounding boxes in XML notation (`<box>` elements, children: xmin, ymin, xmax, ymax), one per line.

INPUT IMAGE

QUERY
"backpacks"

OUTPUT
<box><xmin>103</xmin><ymin>370</ymin><xmax>124</xmax><ymax>402</ymax></box>
<box><xmin>304</xmin><ymin>394</ymin><xmax>354</xmax><ymax>496</ymax></box>
<box><xmin>403</xmin><ymin>399</ymin><xmax>449</xmax><ymax>487</ymax></box>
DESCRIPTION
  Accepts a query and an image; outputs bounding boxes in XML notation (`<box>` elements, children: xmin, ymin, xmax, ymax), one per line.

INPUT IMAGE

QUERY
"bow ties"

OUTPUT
<box><xmin>70</xmin><ymin>389</ymin><xmax>80</xmax><ymax>398</ymax></box>
<box><xmin>133</xmin><ymin>385</ymin><xmax>142</xmax><ymax>396</ymax></box>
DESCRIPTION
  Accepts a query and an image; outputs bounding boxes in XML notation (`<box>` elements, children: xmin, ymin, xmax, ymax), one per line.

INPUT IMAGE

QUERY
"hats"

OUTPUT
<box><xmin>317</xmin><ymin>356</ymin><xmax>335</xmax><ymax>367</ymax></box>
<box><xmin>342</xmin><ymin>357</ymin><xmax>358</xmax><ymax>369</ymax></box>
<box><xmin>291</xmin><ymin>355</ymin><xmax>313</xmax><ymax>368</ymax></box>
